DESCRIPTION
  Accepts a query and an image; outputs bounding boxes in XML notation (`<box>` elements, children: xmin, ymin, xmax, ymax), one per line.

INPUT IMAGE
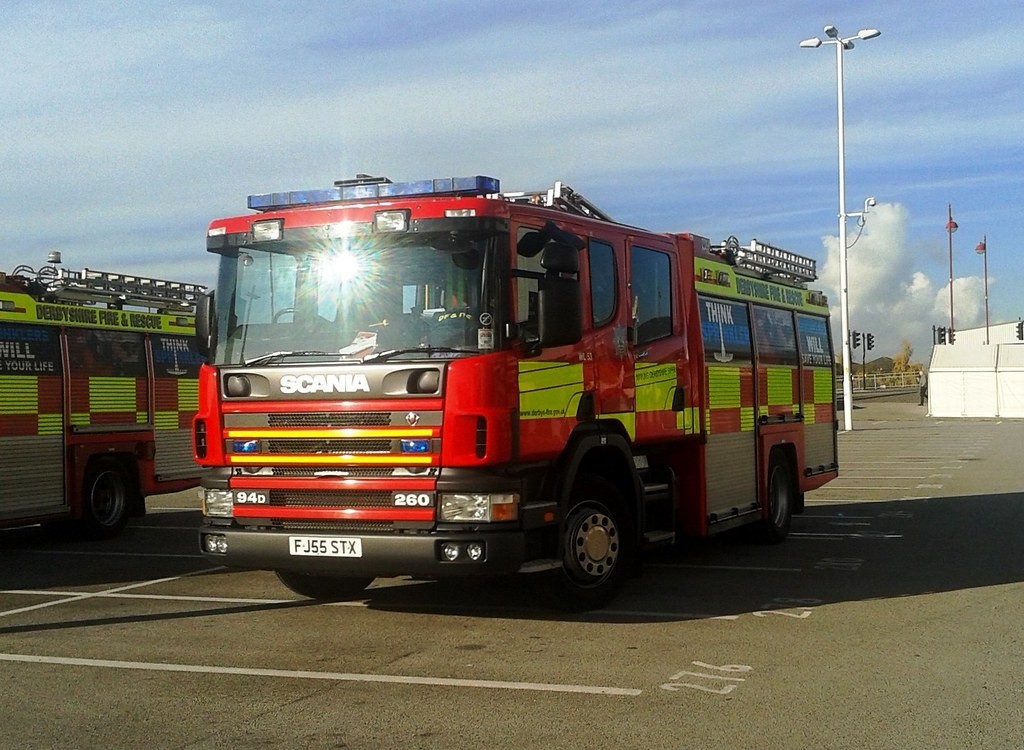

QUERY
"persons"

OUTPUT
<box><xmin>917</xmin><ymin>370</ymin><xmax>928</xmax><ymax>406</ymax></box>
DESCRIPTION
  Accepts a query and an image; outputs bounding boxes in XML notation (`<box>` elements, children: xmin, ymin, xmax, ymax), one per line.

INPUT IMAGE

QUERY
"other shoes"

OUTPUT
<box><xmin>918</xmin><ymin>404</ymin><xmax>923</xmax><ymax>406</ymax></box>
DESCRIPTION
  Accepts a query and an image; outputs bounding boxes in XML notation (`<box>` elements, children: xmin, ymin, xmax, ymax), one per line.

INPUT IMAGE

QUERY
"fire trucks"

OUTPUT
<box><xmin>190</xmin><ymin>174</ymin><xmax>841</xmax><ymax>610</ymax></box>
<box><xmin>0</xmin><ymin>250</ymin><xmax>209</xmax><ymax>544</ymax></box>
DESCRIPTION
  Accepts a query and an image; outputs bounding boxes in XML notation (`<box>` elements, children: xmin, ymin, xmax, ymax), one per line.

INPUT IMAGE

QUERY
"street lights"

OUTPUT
<box><xmin>947</xmin><ymin>221</ymin><xmax>958</xmax><ymax>346</ymax></box>
<box><xmin>799</xmin><ymin>25</ymin><xmax>882</xmax><ymax>431</ymax></box>
<box><xmin>975</xmin><ymin>241</ymin><xmax>990</xmax><ymax>344</ymax></box>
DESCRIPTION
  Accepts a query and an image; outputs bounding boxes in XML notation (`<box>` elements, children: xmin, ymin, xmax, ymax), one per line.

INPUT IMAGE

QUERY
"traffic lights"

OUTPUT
<box><xmin>949</xmin><ymin>328</ymin><xmax>956</xmax><ymax>344</ymax></box>
<box><xmin>852</xmin><ymin>330</ymin><xmax>860</xmax><ymax>349</ymax></box>
<box><xmin>938</xmin><ymin>327</ymin><xmax>946</xmax><ymax>345</ymax></box>
<box><xmin>867</xmin><ymin>333</ymin><xmax>875</xmax><ymax>350</ymax></box>
<box><xmin>1015</xmin><ymin>323</ymin><xmax>1023</xmax><ymax>340</ymax></box>
